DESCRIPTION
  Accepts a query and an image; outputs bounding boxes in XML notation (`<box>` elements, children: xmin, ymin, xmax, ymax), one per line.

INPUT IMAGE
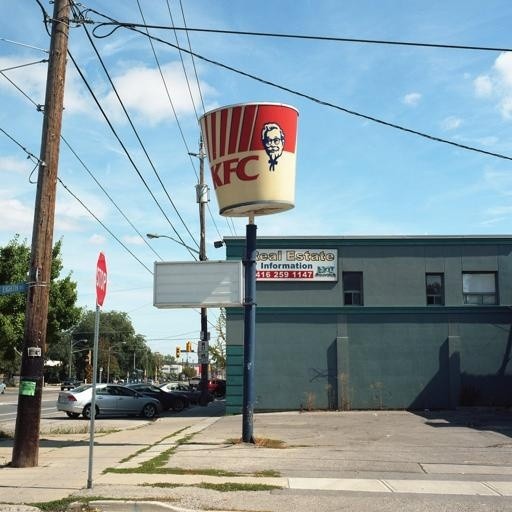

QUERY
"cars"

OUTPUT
<box><xmin>56</xmin><ymin>378</ymin><xmax>225</xmax><ymax>419</ymax></box>
<box><xmin>0</xmin><ymin>383</ymin><xmax>6</xmax><ymax>394</ymax></box>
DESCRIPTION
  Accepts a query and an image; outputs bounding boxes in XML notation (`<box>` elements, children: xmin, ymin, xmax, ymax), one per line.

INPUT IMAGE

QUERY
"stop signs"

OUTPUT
<box><xmin>96</xmin><ymin>251</ymin><xmax>107</xmax><ymax>307</ymax></box>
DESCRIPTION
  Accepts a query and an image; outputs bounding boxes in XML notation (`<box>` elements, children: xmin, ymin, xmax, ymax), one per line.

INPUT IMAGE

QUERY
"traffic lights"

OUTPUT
<box><xmin>176</xmin><ymin>346</ymin><xmax>180</xmax><ymax>357</ymax></box>
<box><xmin>186</xmin><ymin>341</ymin><xmax>192</xmax><ymax>352</ymax></box>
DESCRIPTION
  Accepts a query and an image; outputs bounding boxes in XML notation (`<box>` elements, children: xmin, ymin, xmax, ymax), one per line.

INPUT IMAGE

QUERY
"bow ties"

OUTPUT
<box><xmin>268</xmin><ymin>159</ymin><xmax>279</xmax><ymax>172</ymax></box>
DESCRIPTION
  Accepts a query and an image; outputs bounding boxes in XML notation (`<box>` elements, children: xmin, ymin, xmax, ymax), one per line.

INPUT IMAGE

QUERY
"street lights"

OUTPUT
<box><xmin>107</xmin><ymin>342</ymin><xmax>126</xmax><ymax>383</ymax></box>
<box><xmin>69</xmin><ymin>339</ymin><xmax>87</xmax><ymax>378</ymax></box>
<box><xmin>146</xmin><ymin>232</ymin><xmax>200</xmax><ymax>254</ymax></box>
